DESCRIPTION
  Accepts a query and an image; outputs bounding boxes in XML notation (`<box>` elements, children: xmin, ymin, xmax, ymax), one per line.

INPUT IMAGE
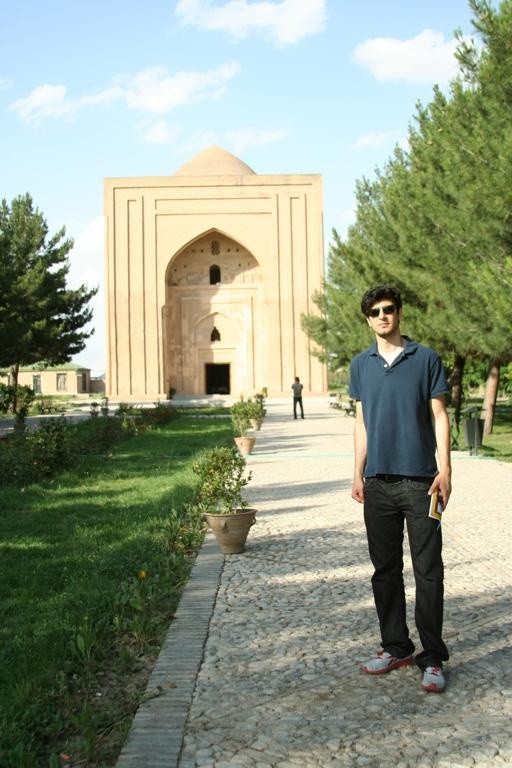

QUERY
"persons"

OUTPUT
<box><xmin>346</xmin><ymin>284</ymin><xmax>457</xmax><ymax>693</ymax></box>
<box><xmin>291</xmin><ymin>377</ymin><xmax>305</xmax><ymax>419</ymax></box>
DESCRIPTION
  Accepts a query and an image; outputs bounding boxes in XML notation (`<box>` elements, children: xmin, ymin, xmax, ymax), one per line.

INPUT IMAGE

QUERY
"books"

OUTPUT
<box><xmin>428</xmin><ymin>486</ymin><xmax>443</xmax><ymax>531</ymax></box>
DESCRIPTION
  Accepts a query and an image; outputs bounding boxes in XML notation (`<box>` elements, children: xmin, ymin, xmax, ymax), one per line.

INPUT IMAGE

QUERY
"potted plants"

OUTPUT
<box><xmin>191</xmin><ymin>447</ymin><xmax>257</xmax><ymax>554</ymax></box>
<box><xmin>12</xmin><ymin>403</ymin><xmax>26</xmax><ymax>433</ymax></box>
<box><xmin>89</xmin><ymin>402</ymin><xmax>99</xmax><ymax>420</ymax></box>
<box><xmin>229</xmin><ymin>393</ymin><xmax>266</xmax><ymax>454</ymax></box>
<box><xmin>100</xmin><ymin>396</ymin><xmax>108</xmax><ymax>414</ymax></box>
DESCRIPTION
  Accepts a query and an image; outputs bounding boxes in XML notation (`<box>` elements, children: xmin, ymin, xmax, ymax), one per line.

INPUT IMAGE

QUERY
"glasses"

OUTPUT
<box><xmin>368</xmin><ymin>302</ymin><xmax>395</xmax><ymax>317</ymax></box>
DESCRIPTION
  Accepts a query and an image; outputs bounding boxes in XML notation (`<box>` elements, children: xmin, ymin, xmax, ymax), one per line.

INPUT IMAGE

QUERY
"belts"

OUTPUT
<box><xmin>377</xmin><ymin>475</ymin><xmax>415</xmax><ymax>482</ymax></box>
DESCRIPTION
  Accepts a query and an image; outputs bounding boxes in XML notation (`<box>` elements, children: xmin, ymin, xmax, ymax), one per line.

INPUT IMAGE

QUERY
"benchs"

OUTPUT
<box><xmin>38</xmin><ymin>399</ymin><xmax>56</xmax><ymax>414</ymax></box>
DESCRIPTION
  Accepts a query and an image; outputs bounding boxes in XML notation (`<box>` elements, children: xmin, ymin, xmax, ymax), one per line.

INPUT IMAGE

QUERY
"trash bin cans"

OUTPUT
<box><xmin>457</xmin><ymin>404</ymin><xmax>485</xmax><ymax>454</ymax></box>
<box><xmin>328</xmin><ymin>391</ymin><xmax>340</xmax><ymax>404</ymax></box>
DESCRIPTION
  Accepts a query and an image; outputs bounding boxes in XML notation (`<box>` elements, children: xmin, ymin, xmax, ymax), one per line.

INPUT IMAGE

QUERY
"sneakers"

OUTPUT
<box><xmin>363</xmin><ymin>648</ymin><xmax>413</xmax><ymax>675</ymax></box>
<box><xmin>421</xmin><ymin>666</ymin><xmax>446</xmax><ymax>692</ymax></box>
